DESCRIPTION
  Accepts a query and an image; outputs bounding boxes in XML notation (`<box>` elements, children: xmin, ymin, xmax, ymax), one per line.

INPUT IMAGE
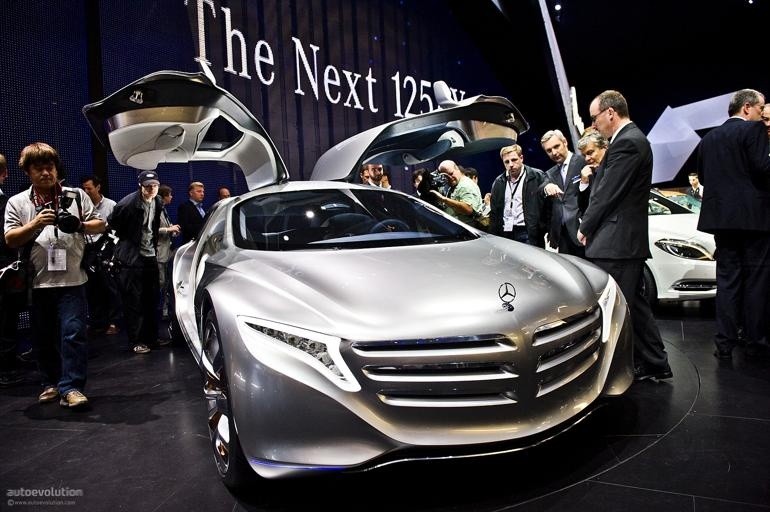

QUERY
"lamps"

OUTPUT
<box><xmin>322</xmin><ymin>211</ymin><xmax>377</xmax><ymax>238</ymax></box>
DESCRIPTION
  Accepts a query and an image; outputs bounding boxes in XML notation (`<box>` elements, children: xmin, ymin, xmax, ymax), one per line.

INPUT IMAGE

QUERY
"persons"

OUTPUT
<box><xmin>686</xmin><ymin>172</ymin><xmax>703</xmax><ymax>200</ymax></box>
<box><xmin>1</xmin><ymin>126</ymin><xmax>610</xmax><ymax>388</ymax></box>
<box><xmin>576</xmin><ymin>89</ymin><xmax>674</xmax><ymax>385</ymax></box>
<box><xmin>1</xmin><ymin>139</ymin><xmax>106</xmax><ymax>410</ymax></box>
<box><xmin>694</xmin><ymin>86</ymin><xmax>770</xmax><ymax>365</ymax></box>
<box><xmin>762</xmin><ymin>103</ymin><xmax>770</xmax><ymax>133</ymax></box>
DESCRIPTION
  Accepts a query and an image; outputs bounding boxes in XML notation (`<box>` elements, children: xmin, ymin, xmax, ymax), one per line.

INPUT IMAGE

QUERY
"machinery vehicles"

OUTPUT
<box><xmin>80</xmin><ymin>67</ymin><xmax>636</xmax><ymax>493</ymax></box>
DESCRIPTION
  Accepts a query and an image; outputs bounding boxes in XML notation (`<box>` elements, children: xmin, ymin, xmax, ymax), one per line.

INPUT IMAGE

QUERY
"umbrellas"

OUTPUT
<box><xmin>634</xmin><ymin>366</ymin><xmax>673</xmax><ymax>379</ymax></box>
<box><xmin>714</xmin><ymin>347</ymin><xmax>732</xmax><ymax>360</ymax></box>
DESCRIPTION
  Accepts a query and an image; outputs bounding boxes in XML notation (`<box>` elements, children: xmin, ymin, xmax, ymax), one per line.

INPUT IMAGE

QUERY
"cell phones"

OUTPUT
<box><xmin>417</xmin><ymin>168</ymin><xmax>449</xmax><ymax>194</ymax></box>
<box><xmin>88</xmin><ymin>240</ymin><xmax>121</xmax><ymax>278</ymax></box>
<box><xmin>49</xmin><ymin>190</ymin><xmax>80</xmax><ymax>234</ymax></box>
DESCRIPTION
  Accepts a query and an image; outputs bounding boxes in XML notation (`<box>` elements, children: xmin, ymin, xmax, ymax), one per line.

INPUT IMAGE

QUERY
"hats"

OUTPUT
<box><xmin>648</xmin><ymin>189</ymin><xmax>701</xmax><ymax>215</ymax></box>
<box><xmin>639</xmin><ymin>189</ymin><xmax>717</xmax><ymax>307</ymax></box>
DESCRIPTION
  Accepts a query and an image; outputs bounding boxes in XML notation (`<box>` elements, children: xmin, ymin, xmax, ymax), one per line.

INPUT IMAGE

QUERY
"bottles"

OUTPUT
<box><xmin>138</xmin><ymin>171</ymin><xmax>159</xmax><ymax>187</ymax></box>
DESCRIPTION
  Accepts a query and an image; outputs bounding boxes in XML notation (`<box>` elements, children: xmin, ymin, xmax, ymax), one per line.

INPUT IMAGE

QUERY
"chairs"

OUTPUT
<box><xmin>60</xmin><ymin>389</ymin><xmax>88</xmax><ymax>407</ymax></box>
<box><xmin>133</xmin><ymin>344</ymin><xmax>150</xmax><ymax>354</ymax></box>
<box><xmin>0</xmin><ymin>373</ymin><xmax>38</xmax><ymax>384</ymax></box>
<box><xmin>39</xmin><ymin>389</ymin><xmax>58</xmax><ymax>402</ymax></box>
